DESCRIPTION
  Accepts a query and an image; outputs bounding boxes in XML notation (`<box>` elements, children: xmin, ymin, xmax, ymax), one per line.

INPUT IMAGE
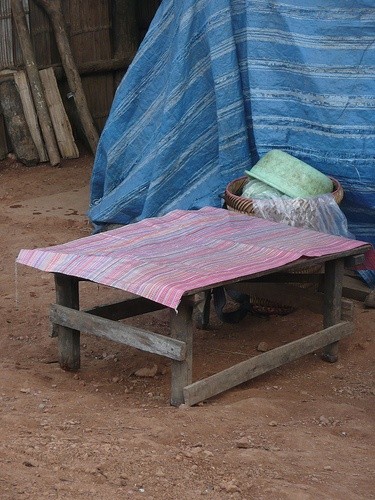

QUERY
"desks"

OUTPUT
<box><xmin>17</xmin><ymin>202</ymin><xmax>371</xmax><ymax>409</ymax></box>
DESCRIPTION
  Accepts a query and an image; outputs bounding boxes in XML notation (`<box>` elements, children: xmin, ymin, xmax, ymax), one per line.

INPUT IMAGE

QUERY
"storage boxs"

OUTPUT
<box><xmin>223</xmin><ymin>174</ymin><xmax>345</xmax><ymax>319</ymax></box>
